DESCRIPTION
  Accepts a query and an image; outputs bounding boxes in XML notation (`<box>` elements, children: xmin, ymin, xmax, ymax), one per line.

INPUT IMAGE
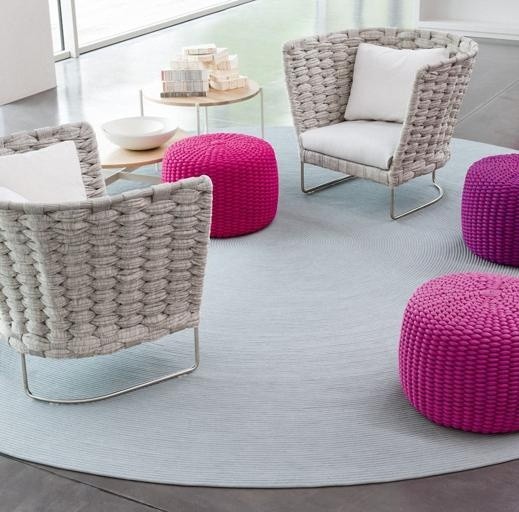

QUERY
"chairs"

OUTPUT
<box><xmin>0</xmin><ymin>121</ymin><xmax>213</xmax><ymax>403</ymax></box>
<box><xmin>282</xmin><ymin>27</ymin><xmax>480</xmax><ymax>220</ymax></box>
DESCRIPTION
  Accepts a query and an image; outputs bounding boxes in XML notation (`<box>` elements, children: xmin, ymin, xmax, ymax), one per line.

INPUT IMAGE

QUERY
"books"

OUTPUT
<box><xmin>155</xmin><ymin>41</ymin><xmax>254</xmax><ymax>101</ymax></box>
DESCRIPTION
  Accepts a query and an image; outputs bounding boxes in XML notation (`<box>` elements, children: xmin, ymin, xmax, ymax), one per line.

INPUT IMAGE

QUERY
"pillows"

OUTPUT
<box><xmin>0</xmin><ymin>142</ymin><xmax>89</xmax><ymax>204</ymax></box>
<box><xmin>344</xmin><ymin>42</ymin><xmax>450</xmax><ymax>125</ymax></box>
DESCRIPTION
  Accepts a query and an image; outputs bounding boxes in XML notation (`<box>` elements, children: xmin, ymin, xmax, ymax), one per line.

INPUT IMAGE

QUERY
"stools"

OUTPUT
<box><xmin>399</xmin><ymin>272</ymin><xmax>519</xmax><ymax>433</ymax></box>
<box><xmin>460</xmin><ymin>154</ymin><xmax>519</xmax><ymax>266</ymax></box>
<box><xmin>164</xmin><ymin>133</ymin><xmax>279</xmax><ymax>239</ymax></box>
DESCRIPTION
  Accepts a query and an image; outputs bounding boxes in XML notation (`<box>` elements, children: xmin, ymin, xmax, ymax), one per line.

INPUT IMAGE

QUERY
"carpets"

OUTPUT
<box><xmin>0</xmin><ymin>125</ymin><xmax>519</xmax><ymax>488</ymax></box>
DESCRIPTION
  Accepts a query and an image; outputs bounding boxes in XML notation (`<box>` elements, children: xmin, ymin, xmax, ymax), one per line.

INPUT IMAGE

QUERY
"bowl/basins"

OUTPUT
<box><xmin>102</xmin><ymin>116</ymin><xmax>181</xmax><ymax>151</ymax></box>
